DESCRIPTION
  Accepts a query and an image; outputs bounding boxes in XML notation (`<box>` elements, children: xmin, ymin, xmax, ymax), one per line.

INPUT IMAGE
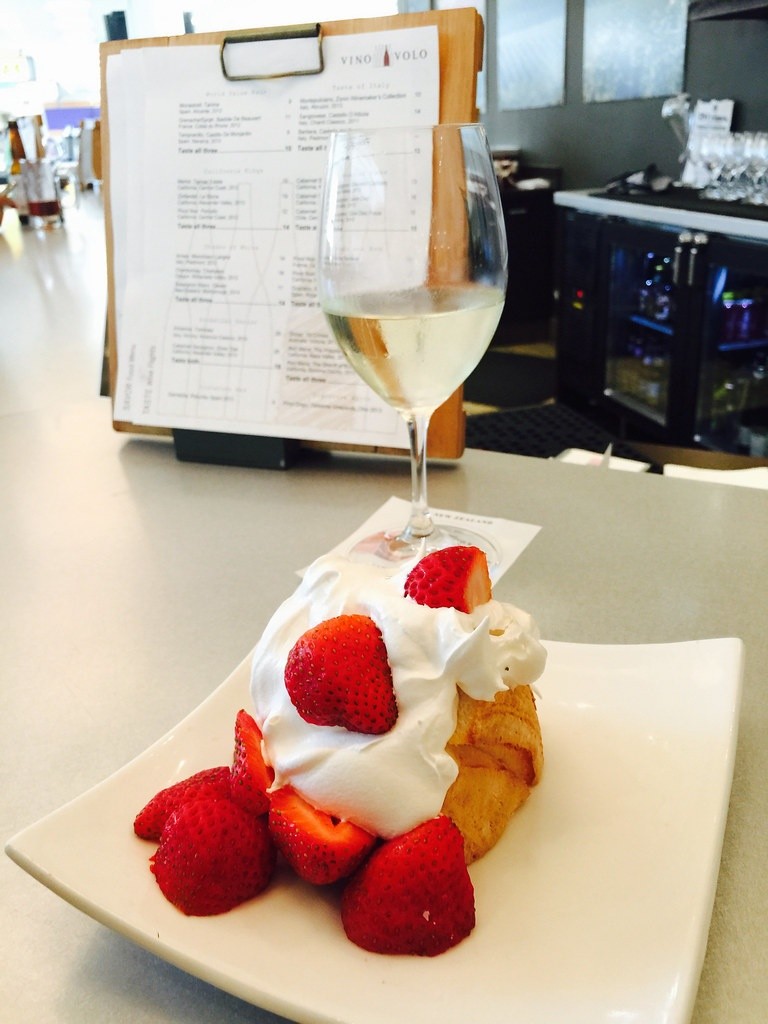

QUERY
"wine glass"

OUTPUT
<box><xmin>698</xmin><ymin>129</ymin><xmax>768</xmax><ymax>206</ymax></box>
<box><xmin>319</xmin><ymin>120</ymin><xmax>511</xmax><ymax>595</ymax></box>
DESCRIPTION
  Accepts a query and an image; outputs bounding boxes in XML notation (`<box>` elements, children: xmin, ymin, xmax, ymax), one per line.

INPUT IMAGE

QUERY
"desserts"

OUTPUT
<box><xmin>246</xmin><ymin>544</ymin><xmax>545</xmax><ymax>865</ymax></box>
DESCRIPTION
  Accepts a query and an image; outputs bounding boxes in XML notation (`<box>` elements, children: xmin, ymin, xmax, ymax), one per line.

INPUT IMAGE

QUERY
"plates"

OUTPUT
<box><xmin>6</xmin><ymin>580</ymin><xmax>744</xmax><ymax>1023</ymax></box>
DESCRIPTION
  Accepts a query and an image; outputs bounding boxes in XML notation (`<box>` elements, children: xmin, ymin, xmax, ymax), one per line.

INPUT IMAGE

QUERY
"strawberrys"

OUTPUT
<box><xmin>129</xmin><ymin>708</ymin><xmax>475</xmax><ymax>957</ymax></box>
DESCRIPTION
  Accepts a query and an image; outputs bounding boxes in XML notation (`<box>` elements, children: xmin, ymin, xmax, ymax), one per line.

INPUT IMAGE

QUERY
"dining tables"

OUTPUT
<box><xmin>0</xmin><ymin>181</ymin><xmax>768</xmax><ymax>1024</ymax></box>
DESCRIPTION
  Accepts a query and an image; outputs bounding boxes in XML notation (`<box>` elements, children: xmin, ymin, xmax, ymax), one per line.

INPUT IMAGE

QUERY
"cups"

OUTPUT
<box><xmin>18</xmin><ymin>157</ymin><xmax>81</xmax><ymax>231</ymax></box>
